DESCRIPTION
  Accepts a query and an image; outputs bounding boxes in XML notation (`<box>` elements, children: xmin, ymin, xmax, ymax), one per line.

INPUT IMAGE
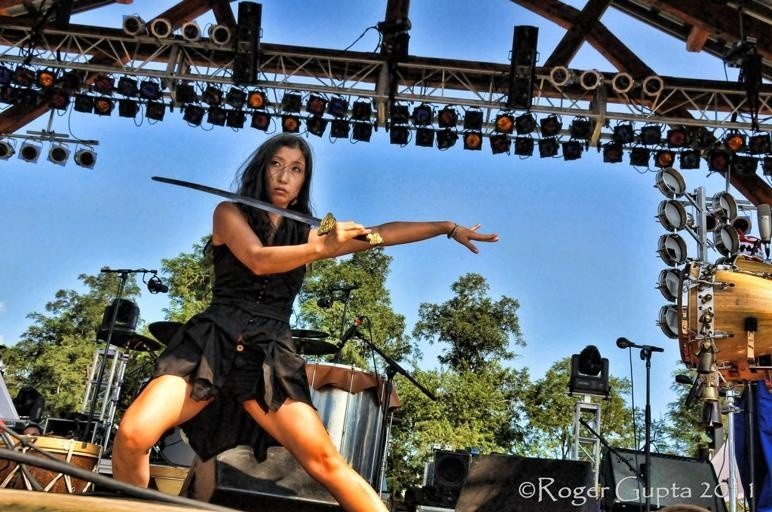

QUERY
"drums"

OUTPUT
<box><xmin>0</xmin><ymin>434</ymin><xmax>103</xmax><ymax>496</ymax></box>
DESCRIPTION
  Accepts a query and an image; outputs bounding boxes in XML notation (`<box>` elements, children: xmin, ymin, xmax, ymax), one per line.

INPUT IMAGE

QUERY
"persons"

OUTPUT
<box><xmin>25</xmin><ymin>423</ymin><xmax>42</xmax><ymax>439</ymax></box>
<box><xmin>109</xmin><ymin>134</ymin><xmax>498</xmax><ymax>511</ymax></box>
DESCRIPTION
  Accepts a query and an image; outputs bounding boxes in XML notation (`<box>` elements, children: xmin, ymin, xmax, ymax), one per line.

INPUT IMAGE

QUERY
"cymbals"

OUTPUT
<box><xmin>292</xmin><ymin>328</ymin><xmax>330</xmax><ymax>338</ymax></box>
<box><xmin>295</xmin><ymin>338</ymin><xmax>338</xmax><ymax>355</ymax></box>
<box><xmin>149</xmin><ymin>322</ymin><xmax>185</xmax><ymax>345</ymax></box>
<box><xmin>99</xmin><ymin>331</ymin><xmax>162</xmax><ymax>351</ymax></box>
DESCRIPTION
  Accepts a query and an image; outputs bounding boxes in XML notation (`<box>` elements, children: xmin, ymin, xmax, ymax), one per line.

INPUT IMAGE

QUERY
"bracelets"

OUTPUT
<box><xmin>446</xmin><ymin>224</ymin><xmax>461</xmax><ymax>240</ymax></box>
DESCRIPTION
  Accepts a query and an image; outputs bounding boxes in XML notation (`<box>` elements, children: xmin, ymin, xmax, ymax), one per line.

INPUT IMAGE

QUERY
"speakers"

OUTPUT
<box><xmin>433</xmin><ymin>451</ymin><xmax>473</xmax><ymax>503</ymax></box>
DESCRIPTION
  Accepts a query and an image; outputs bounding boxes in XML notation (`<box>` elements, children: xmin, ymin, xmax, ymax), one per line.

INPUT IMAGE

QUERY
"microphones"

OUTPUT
<box><xmin>336</xmin><ymin>316</ymin><xmax>364</xmax><ymax>349</ymax></box>
<box><xmin>317</xmin><ymin>296</ymin><xmax>332</xmax><ymax>308</ymax></box>
<box><xmin>148</xmin><ymin>275</ymin><xmax>168</xmax><ymax>294</ymax></box>
<box><xmin>616</xmin><ymin>337</ymin><xmax>664</xmax><ymax>352</ymax></box>
